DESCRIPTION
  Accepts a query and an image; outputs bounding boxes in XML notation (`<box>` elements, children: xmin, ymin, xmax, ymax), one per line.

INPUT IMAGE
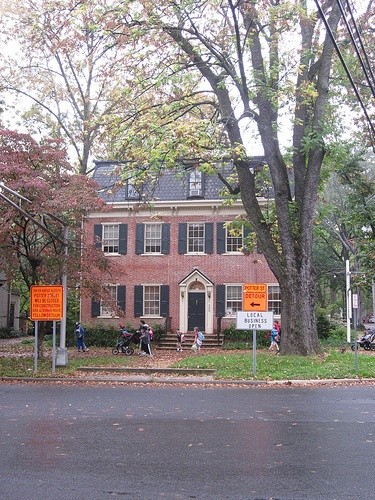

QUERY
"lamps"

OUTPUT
<box><xmin>181</xmin><ymin>291</ymin><xmax>184</xmax><ymax>297</ymax></box>
<box><xmin>207</xmin><ymin>291</ymin><xmax>211</xmax><ymax>297</ymax></box>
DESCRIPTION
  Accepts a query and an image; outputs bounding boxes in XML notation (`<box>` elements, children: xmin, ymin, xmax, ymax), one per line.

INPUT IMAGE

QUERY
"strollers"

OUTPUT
<box><xmin>351</xmin><ymin>328</ymin><xmax>375</xmax><ymax>351</ymax></box>
<box><xmin>112</xmin><ymin>332</ymin><xmax>142</xmax><ymax>356</ymax></box>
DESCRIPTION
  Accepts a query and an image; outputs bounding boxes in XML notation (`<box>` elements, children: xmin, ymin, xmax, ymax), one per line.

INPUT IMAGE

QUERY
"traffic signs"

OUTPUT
<box><xmin>241</xmin><ymin>292</ymin><xmax>268</xmax><ymax>312</ymax></box>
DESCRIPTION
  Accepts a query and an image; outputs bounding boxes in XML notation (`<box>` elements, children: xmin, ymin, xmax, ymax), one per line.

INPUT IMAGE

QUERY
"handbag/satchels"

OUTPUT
<box><xmin>274</xmin><ymin>334</ymin><xmax>279</xmax><ymax>341</ymax></box>
<box><xmin>190</xmin><ymin>343</ymin><xmax>197</xmax><ymax>353</ymax></box>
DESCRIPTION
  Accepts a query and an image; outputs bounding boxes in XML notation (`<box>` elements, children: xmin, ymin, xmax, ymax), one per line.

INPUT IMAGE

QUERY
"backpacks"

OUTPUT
<box><xmin>197</xmin><ymin>332</ymin><xmax>204</xmax><ymax>342</ymax></box>
<box><xmin>180</xmin><ymin>334</ymin><xmax>185</xmax><ymax>343</ymax></box>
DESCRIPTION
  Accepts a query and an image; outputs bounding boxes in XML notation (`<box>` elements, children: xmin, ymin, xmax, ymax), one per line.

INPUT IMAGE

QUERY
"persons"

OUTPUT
<box><xmin>74</xmin><ymin>322</ymin><xmax>86</xmax><ymax>352</ymax></box>
<box><xmin>175</xmin><ymin>328</ymin><xmax>184</xmax><ymax>352</ymax></box>
<box><xmin>265</xmin><ymin>320</ymin><xmax>281</xmax><ymax>352</ymax></box>
<box><xmin>194</xmin><ymin>327</ymin><xmax>202</xmax><ymax>356</ymax></box>
<box><xmin>119</xmin><ymin>320</ymin><xmax>154</xmax><ymax>357</ymax></box>
